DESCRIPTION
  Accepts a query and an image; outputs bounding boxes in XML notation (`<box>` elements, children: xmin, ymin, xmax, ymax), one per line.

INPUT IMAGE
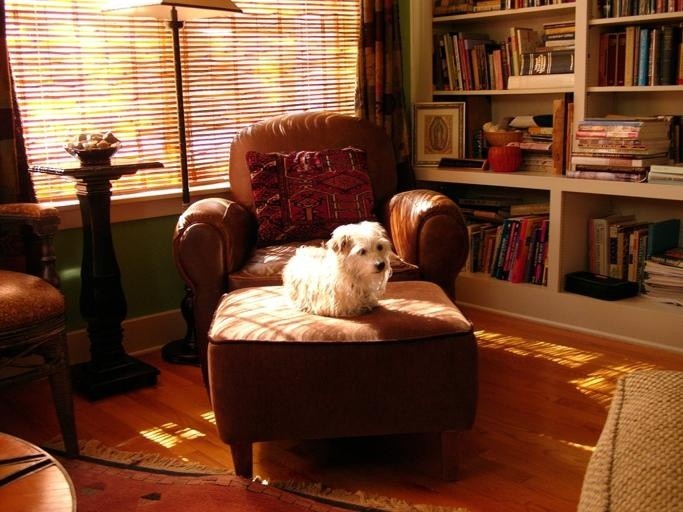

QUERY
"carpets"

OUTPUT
<box><xmin>40</xmin><ymin>433</ymin><xmax>470</xmax><ymax>510</ymax></box>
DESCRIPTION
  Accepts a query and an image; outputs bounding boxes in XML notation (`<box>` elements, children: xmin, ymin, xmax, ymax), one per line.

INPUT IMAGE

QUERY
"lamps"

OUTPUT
<box><xmin>98</xmin><ymin>0</ymin><xmax>243</xmax><ymax>365</ymax></box>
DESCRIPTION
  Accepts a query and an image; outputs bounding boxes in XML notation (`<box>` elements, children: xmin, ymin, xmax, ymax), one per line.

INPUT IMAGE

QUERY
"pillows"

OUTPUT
<box><xmin>244</xmin><ymin>145</ymin><xmax>377</xmax><ymax>250</ymax></box>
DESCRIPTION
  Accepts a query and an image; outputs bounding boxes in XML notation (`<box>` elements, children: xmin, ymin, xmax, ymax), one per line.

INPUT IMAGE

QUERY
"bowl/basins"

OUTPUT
<box><xmin>64</xmin><ymin>142</ymin><xmax>122</xmax><ymax>165</ymax></box>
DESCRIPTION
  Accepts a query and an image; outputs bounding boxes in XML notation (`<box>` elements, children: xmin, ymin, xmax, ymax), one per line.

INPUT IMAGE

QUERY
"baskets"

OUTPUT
<box><xmin>486</xmin><ymin>131</ymin><xmax>523</xmax><ymax>146</ymax></box>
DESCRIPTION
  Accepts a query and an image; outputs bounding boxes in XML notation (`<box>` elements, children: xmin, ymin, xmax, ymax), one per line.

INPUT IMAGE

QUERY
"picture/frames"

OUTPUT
<box><xmin>411</xmin><ymin>102</ymin><xmax>466</xmax><ymax>168</ymax></box>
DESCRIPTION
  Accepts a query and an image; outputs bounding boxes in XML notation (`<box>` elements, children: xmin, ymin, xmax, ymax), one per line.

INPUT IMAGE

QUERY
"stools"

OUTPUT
<box><xmin>206</xmin><ymin>280</ymin><xmax>478</xmax><ymax>481</ymax></box>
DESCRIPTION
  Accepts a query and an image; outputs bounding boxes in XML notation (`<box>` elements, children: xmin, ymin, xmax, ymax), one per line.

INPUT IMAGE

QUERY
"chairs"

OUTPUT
<box><xmin>408</xmin><ymin>0</ymin><xmax>683</xmax><ymax>360</ymax></box>
<box><xmin>0</xmin><ymin>202</ymin><xmax>81</xmax><ymax>460</ymax></box>
<box><xmin>172</xmin><ymin>110</ymin><xmax>469</xmax><ymax>409</ymax></box>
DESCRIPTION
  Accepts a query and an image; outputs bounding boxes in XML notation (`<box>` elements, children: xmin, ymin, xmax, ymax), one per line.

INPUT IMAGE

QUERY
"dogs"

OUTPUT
<box><xmin>281</xmin><ymin>220</ymin><xmax>395</xmax><ymax>319</ymax></box>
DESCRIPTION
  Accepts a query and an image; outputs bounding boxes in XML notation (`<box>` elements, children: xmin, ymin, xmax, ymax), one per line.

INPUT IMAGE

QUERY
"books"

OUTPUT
<box><xmin>509</xmin><ymin>95</ymin><xmax>683</xmax><ymax>184</ymax></box>
<box><xmin>432</xmin><ymin>0</ymin><xmax>683</xmax><ymax>91</ymax></box>
<box><xmin>563</xmin><ymin>211</ymin><xmax>683</xmax><ymax>307</ymax></box>
<box><xmin>456</xmin><ymin>196</ymin><xmax>549</xmax><ymax>286</ymax></box>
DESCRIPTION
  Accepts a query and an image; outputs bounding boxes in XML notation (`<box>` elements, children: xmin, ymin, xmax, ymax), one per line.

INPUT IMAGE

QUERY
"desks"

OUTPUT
<box><xmin>26</xmin><ymin>158</ymin><xmax>164</xmax><ymax>403</ymax></box>
<box><xmin>0</xmin><ymin>429</ymin><xmax>77</xmax><ymax>511</ymax></box>
